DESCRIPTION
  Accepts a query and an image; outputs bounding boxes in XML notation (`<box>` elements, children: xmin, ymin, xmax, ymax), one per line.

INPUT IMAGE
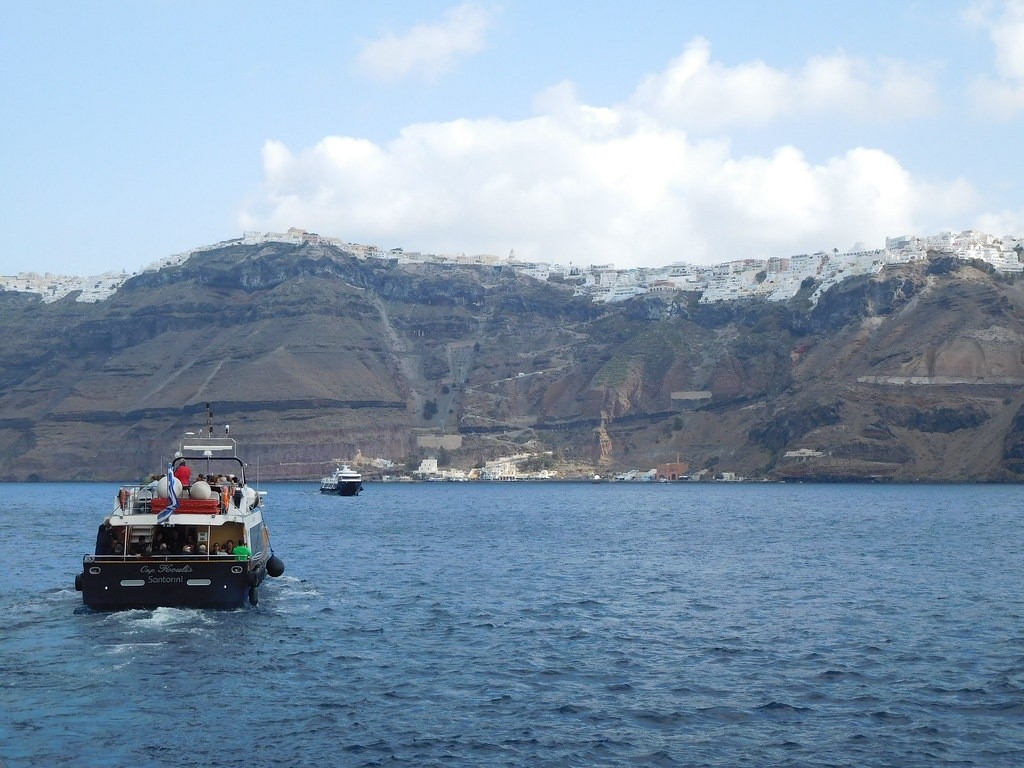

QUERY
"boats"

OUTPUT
<box><xmin>75</xmin><ymin>401</ymin><xmax>286</xmax><ymax>608</ymax></box>
<box><xmin>320</xmin><ymin>460</ymin><xmax>363</xmax><ymax>496</ymax></box>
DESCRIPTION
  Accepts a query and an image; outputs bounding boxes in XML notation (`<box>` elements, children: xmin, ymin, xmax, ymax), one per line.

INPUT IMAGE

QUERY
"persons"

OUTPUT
<box><xmin>142</xmin><ymin>458</ymin><xmax>242</xmax><ymax>502</ymax></box>
<box><xmin>109</xmin><ymin>532</ymin><xmax>252</xmax><ymax>562</ymax></box>
